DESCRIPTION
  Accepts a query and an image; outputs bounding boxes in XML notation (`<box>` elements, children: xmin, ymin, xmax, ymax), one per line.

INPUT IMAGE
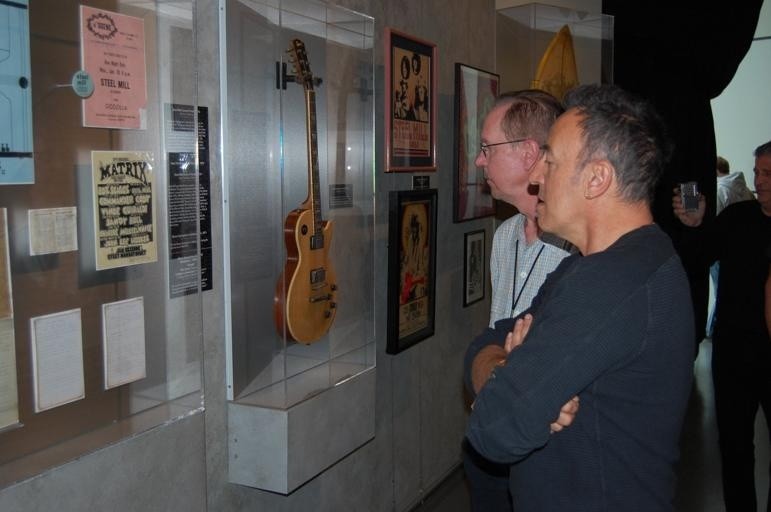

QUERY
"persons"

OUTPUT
<box><xmin>461</xmin><ymin>84</ymin><xmax>696</xmax><ymax>512</ymax></box>
<box><xmin>474</xmin><ymin>88</ymin><xmax>580</xmax><ymax>329</ymax></box>
<box><xmin>672</xmin><ymin>142</ymin><xmax>771</xmax><ymax>512</ymax></box>
<box><xmin>710</xmin><ymin>157</ymin><xmax>757</xmax><ymax>336</ymax></box>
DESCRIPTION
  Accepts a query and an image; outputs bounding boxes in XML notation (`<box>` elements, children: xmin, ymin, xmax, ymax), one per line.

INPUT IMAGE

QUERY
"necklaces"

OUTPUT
<box><xmin>510</xmin><ymin>238</ymin><xmax>546</xmax><ymax>319</ymax></box>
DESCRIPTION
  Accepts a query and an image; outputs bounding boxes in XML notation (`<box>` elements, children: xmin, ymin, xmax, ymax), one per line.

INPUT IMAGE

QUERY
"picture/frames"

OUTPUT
<box><xmin>462</xmin><ymin>228</ymin><xmax>486</xmax><ymax>307</ymax></box>
<box><xmin>453</xmin><ymin>62</ymin><xmax>501</xmax><ymax>224</ymax></box>
<box><xmin>388</xmin><ymin>188</ymin><xmax>437</xmax><ymax>356</ymax></box>
<box><xmin>384</xmin><ymin>25</ymin><xmax>438</xmax><ymax>173</ymax></box>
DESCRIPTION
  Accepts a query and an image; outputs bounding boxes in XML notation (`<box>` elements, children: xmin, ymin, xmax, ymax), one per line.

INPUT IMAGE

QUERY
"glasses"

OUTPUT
<box><xmin>479</xmin><ymin>138</ymin><xmax>528</xmax><ymax>156</ymax></box>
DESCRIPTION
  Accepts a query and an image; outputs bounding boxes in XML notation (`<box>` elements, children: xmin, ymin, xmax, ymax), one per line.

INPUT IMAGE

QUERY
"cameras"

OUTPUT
<box><xmin>680</xmin><ymin>182</ymin><xmax>699</xmax><ymax>210</ymax></box>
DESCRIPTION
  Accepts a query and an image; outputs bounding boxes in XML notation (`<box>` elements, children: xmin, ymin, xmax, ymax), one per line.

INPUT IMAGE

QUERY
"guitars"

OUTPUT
<box><xmin>274</xmin><ymin>38</ymin><xmax>339</xmax><ymax>345</ymax></box>
